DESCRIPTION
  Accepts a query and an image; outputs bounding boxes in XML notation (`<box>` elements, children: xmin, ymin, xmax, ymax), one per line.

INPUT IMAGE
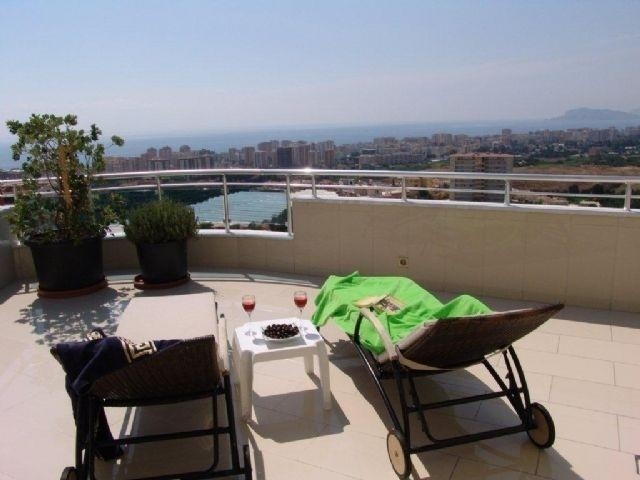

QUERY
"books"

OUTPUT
<box><xmin>354</xmin><ymin>291</ymin><xmax>405</xmax><ymax>315</ymax></box>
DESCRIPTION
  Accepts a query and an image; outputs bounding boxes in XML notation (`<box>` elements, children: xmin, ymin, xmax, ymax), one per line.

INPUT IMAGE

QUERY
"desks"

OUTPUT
<box><xmin>232</xmin><ymin>317</ymin><xmax>331</xmax><ymax>424</ymax></box>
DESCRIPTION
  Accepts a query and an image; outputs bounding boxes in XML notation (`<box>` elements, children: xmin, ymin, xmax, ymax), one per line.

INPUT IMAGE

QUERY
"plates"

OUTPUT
<box><xmin>261</xmin><ymin>323</ymin><xmax>301</xmax><ymax>342</ymax></box>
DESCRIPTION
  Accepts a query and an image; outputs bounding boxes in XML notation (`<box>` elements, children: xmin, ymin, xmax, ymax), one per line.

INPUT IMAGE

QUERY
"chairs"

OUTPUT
<box><xmin>48</xmin><ymin>291</ymin><xmax>252</xmax><ymax>479</ymax></box>
<box><xmin>313</xmin><ymin>273</ymin><xmax>565</xmax><ymax>480</ymax></box>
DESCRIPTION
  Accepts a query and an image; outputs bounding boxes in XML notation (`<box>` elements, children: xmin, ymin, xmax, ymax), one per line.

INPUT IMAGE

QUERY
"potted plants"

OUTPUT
<box><xmin>121</xmin><ymin>199</ymin><xmax>198</xmax><ymax>289</ymax></box>
<box><xmin>6</xmin><ymin>114</ymin><xmax>125</xmax><ymax>299</ymax></box>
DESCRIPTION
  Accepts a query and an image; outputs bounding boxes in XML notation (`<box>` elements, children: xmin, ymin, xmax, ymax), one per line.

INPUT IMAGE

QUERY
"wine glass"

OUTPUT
<box><xmin>293</xmin><ymin>290</ymin><xmax>308</xmax><ymax>322</ymax></box>
<box><xmin>242</xmin><ymin>295</ymin><xmax>256</xmax><ymax>322</ymax></box>
